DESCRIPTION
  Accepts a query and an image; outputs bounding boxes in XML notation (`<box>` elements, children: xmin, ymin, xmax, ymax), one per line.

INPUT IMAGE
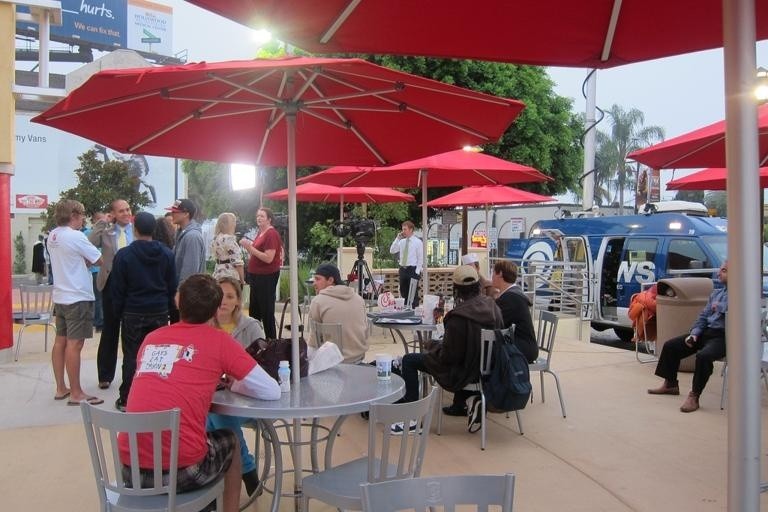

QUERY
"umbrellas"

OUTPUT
<box><xmin>665</xmin><ymin>167</ymin><xmax>767</xmax><ymax>190</ymax></box>
<box><xmin>31</xmin><ymin>54</ymin><xmax>528</xmax><ymax>512</ymax></box>
<box><xmin>187</xmin><ymin>0</ymin><xmax>768</xmax><ymax>512</ymax></box>
<box><xmin>298</xmin><ymin>146</ymin><xmax>557</xmax><ymax>312</ymax></box>
<box><xmin>626</xmin><ymin>100</ymin><xmax>768</xmax><ymax>170</ymax></box>
<box><xmin>417</xmin><ymin>184</ymin><xmax>557</xmax><ymax>254</ymax></box>
<box><xmin>262</xmin><ymin>180</ymin><xmax>416</xmax><ymax>249</ymax></box>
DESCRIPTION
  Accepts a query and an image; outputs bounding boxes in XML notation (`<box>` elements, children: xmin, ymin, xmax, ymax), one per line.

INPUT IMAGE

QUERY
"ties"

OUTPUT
<box><xmin>402</xmin><ymin>239</ymin><xmax>409</xmax><ymax>266</ymax></box>
<box><xmin>118</xmin><ymin>227</ymin><xmax>127</xmax><ymax>249</ymax></box>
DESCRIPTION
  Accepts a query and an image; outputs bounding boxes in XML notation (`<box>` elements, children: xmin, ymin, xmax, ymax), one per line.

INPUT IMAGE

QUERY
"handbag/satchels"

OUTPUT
<box><xmin>258</xmin><ymin>337</ymin><xmax>308</xmax><ymax>380</ymax></box>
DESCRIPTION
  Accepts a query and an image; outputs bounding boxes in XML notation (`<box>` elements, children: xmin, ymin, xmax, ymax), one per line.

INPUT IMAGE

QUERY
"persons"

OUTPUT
<box><xmin>646</xmin><ymin>259</ymin><xmax>728</xmax><ymax>415</ymax></box>
<box><xmin>390</xmin><ymin>221</ymin><xmax>424</xmax><ymax>309</ymax></box>
<box><xmin>389</xmin><ymin>261</ymin><xmax>539</xmax><ymax>435</ymax></box>
<box><xmin>307</xmin><ymin>264</ymin><xmax>371</xmax><ymax>365</ymax></box>
<box><xmin>31</xmin><ymin>199</ymin><xmax>286</xmax><ymax>512</ymax></box>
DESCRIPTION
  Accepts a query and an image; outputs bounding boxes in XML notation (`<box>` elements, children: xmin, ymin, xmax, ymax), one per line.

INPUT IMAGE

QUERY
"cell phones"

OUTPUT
<box><xmin>686</xmin><ymin>335</ymin><xmax>693</xmax><ymax>343</ymax></box>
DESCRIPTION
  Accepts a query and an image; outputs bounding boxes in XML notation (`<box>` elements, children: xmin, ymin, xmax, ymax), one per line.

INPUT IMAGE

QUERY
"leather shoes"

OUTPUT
<box><xmin>242</xmin><ymin>470</ymin><xmax>263</xmax><ymax>497</ymax></box>
<box><xmin>99</xmin><ymin>382</ymin><xmax>110</xmax><ymax>388</ymax></box>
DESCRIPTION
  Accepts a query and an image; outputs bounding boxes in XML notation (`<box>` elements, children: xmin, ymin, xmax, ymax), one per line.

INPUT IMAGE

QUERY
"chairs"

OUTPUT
<box><xmin>630</xmin><ymin>282</ymin><xmax>657</xmax><ymax>363</ymax></box>
<box><xmin>720</xmin><ymin>310</ymin><xmax>768</xmax><ymax>410</ymax></box>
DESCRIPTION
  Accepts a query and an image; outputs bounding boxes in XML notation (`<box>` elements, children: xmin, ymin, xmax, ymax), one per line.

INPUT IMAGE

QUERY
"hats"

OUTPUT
<box><xmin>451</xmin><ymin>252</ymin><xmax>480</xmax><ymax>286</ymax></box>
<box><xmin>313</xmin><ymin>265</ymin><xmax>340</xmax><ymax>279</ymax></box>
<box><xmin>164</xmin><ymin>198</ymin><xmax>196</xmax><ymax>213</ymax></box>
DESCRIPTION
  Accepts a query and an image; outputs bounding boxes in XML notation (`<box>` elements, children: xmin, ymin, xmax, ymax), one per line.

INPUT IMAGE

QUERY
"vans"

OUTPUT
<box><xmin>503</xmin><ymin>198</ymin><xmax>768</xmax><ymax>362</ymax></box>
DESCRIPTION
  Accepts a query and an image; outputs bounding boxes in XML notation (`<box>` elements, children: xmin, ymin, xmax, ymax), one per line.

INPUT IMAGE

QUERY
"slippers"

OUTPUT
<box><xmin>55</xmin><ymin>392</ymin><xmax>70</xmax><ymax>400</ymax></box>
<box><xmin>67</xmin><ymin>397</ymin><xmax>103</xmax><ymax>406</ymax></box>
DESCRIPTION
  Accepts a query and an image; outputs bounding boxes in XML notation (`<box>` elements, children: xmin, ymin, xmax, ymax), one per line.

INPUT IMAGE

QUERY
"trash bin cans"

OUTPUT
<box><xmin>655</xmin><ymin>277</ymin><xmax>714</xmax><ymax>373</ymax></box>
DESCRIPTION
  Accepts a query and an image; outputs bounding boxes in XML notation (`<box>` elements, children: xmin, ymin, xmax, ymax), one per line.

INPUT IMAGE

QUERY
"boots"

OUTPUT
<box><xmin>679</xmin><ymin>392</ymin><xmax>699</xmax><ymax>411</ymax></box>
<box><xmin>648</xmin><ymin>380</ymin><xmax>679</xmax><ymax>394</ymax></box>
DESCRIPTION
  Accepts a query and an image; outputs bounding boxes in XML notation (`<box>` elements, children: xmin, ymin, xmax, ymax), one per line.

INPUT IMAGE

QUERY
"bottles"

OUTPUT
<box><xmin>280</xmin><ymin>393</ymin><xmax>290</xmax><ymax>407</ymax></box>
<box><xmin>278</xmin><ymin>359</ymin><xmax>291</xmax><ymax>393</ymax></box>
<box><xmin>434</xmin><ymin>304</ymin><xmax>444</xmax><ymax>324</ymax></box>
<box><xmin>415</xmin><ymin>307</ymin><xmax>423</xmax><ymax>316</ymax></box>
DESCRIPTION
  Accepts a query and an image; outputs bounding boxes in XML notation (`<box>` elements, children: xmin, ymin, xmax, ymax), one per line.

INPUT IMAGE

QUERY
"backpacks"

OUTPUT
<box><xmin>481</xmin><ymin>326</ymin><xmax>532</xmax><ymax>413</ymax></box>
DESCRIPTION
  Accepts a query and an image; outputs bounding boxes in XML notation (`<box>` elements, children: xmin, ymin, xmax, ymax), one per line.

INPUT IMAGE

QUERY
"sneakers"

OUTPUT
<box><xmin>467</xmin><ymin>396</ymin><xmax>483</xmax><ymax>433</ymax></box>
<box><xmin>116</xmin><ymin>398</ymin><xmax>128</xmax><ymax>412</ymax></box>
<box><xmin>390</xmin><ymin>419</ymin><xmax>423</xmax><ymax>435</ymax></box>
<box><xmin>442</xmin><ymin>405</ymin><xmax>467</xmax><ymax>416</ymax></box>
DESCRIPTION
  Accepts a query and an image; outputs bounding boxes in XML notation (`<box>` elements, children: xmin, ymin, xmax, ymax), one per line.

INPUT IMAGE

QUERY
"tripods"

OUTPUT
<box><xmin>345</xmin><ymin>243</ymin><xmax>379</xmax><ymax>302</ymax></box>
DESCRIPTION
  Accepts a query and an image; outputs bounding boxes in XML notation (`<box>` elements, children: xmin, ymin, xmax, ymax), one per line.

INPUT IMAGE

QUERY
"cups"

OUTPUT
<box><xmin>375</xmin><ymin>354</ymin><xmax>392</xmax><ymax>380</ymax></box>
<box><xmin>395</xmin><ymin>298</ymin><xmax>405</xmax><ymax>310</ymax></box>
<box><xmin>376</xmin><ymin>381</ymin><xmax>393</xmax><ymax>398</ymax></box>
